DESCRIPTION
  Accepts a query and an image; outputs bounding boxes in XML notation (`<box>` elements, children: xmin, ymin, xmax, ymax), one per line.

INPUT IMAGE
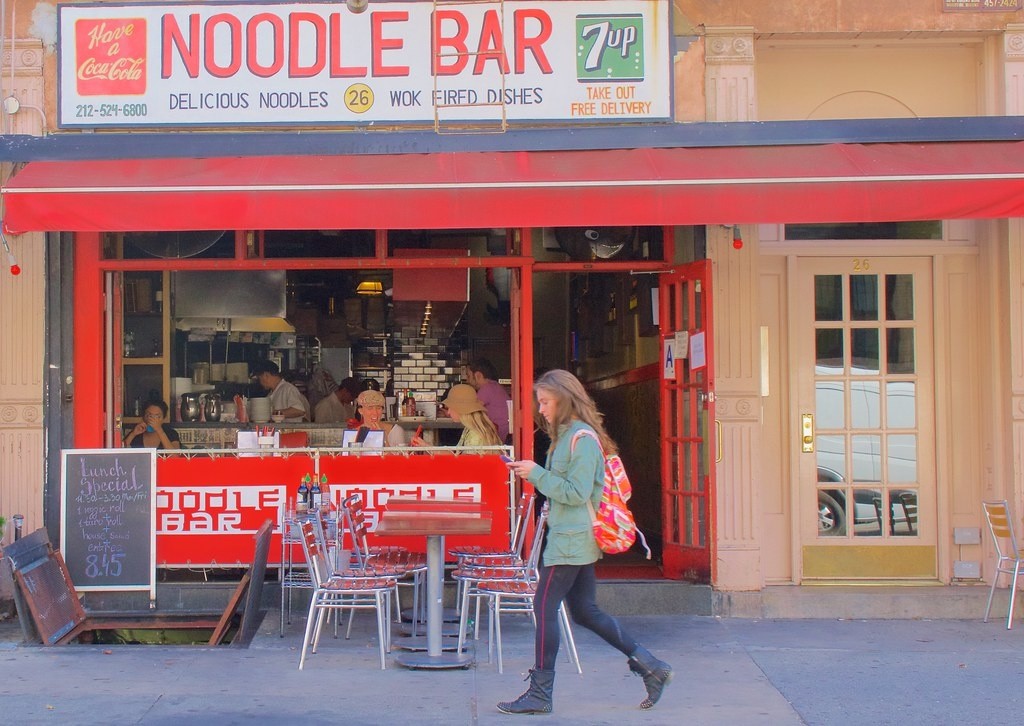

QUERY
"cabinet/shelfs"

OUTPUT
<box><xmin>280</xmin><ymin>496</ymin><xmax>347</xmax><ymax>639</ymax></box>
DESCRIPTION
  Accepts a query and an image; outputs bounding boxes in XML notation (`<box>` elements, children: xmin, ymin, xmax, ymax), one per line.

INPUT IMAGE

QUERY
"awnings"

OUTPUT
<box><xmin>1</xmin><ymin>140</ymin><xmax>1024</xmax><ymax>233</ymax></box>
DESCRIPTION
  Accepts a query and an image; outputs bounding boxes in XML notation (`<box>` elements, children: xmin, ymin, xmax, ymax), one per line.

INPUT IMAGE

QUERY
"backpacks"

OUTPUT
<box><xmin>569</xmin><ymin>428</ymin><xmax>652</xmax><ymax>561</ymax></box>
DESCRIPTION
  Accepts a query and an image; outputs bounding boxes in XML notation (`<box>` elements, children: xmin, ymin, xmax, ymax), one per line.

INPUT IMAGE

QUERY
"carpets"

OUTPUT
<box><xmin>595</xmin><ymin>565</ymin><xmax>664</xmax><ymax>579</ymax></box>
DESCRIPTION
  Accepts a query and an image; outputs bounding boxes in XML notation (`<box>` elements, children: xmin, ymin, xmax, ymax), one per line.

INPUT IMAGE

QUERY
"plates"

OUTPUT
<box><xmin>211</xmin><ymin>362</ymin><xmax>248</xmax><ymax>384</ymax></box>
<box><xmin>249</xmin><ymin>398</ymin><xmax>271</xmax><ymax>423</ymax></box>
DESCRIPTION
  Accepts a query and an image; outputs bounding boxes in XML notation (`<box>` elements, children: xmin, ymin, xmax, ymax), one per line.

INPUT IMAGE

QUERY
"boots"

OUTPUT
<box><xmin>627</xmin><ymin>645</ymin><xmax>673</xmax><ymax>709</ymax></box>
<box><xmin>497</xmin><ymin>668</ymin><xmax>556</xmax><ymax>715</ymax></box>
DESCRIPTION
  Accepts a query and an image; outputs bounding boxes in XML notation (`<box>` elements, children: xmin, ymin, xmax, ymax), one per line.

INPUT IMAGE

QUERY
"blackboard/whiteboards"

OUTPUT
<box><xmin>60</xmin><ymin>447</ymin><xmax>157</xmax><ymax>592</ymax></box>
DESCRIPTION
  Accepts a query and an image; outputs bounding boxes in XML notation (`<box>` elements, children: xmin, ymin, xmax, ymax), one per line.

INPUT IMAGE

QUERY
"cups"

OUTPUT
<box><xmin>236</xmin><ymin>405</ymin><xmax>247</xmax><ymax>423</ymax></box>
<box><xmin>348</xmin><ymin>442</ymin><xmax>363</xmax><ymax>455</ymax></box>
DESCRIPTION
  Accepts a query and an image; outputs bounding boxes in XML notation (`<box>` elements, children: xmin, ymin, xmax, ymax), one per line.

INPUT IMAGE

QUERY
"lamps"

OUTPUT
<box><xmin>356</xmin><ymin>280</ymin><xmax>383</xmax><ymax>295</ymax></box>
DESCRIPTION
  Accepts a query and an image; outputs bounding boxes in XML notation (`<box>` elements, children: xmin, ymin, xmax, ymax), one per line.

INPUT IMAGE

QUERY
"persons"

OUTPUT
<box><xmin>122</xmin><ymin>399</ymin><xmax>180</xmax><ymax>457</ymax></box>
<box><xmin>412</xmin><ymin>384</ymin><xmax>505</xmax><ymax>454</ymax></box>
<box><xmin>356</xmin><ymin>390</ymin><xmax>405</xmax><ymax>447</ymax></box>
<box><xmin>442</xmin><ymin>357</ymin><xmax>513</xmax><ymax>446</ymax></box>
<box><xmin>314</xmin><ymin>377</ymin><xmax>363</xmax><ymax>423</ymax></box>
<box><xmin>250</xmin><ymin>360</ymin><xmax>311</xmax><ymax>423</ymax></box>
<box><xmin>496</xmin><ymin>369</ymin><xmax>676</xmax><ymax>716</ymax></box>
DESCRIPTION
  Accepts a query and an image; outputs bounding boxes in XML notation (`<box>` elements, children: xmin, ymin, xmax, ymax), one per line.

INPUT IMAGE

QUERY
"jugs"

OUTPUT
<box><xmin>204</xmin><ymin>396</ymin><xmax>221</xmax><ymax>421</ymax></box>
<box><xmin>181</xmin><ymin>396</ymin><xmax>199</xmax><ymax>422</ymax></box>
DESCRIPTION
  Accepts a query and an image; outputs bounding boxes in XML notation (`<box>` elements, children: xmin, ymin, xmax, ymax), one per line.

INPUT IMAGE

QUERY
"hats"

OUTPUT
<box><xmin>442</xmin><ymin>384</ymin><xmax>487</xmax><ymax>414</ymax></box>
<box><xmin>250</xmin><ymin>360</ymin><xmax>279</xmax><ymax>378</ymax></box>
<box><xmin>361</xmin><ymin>379</ymin><xmax>379</xmax><ymax>391</ymax></box>
<box><xmin>358</xmin><ymin>390</ymin><xmax>386</xmax><ymax>409</ymax></box>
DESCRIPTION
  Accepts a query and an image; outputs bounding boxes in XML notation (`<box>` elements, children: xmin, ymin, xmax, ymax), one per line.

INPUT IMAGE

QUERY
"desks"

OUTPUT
<box><xmin>375</xmin><ymin>498</ymin><xmax>493</xmax><ymax>670</ymax></box>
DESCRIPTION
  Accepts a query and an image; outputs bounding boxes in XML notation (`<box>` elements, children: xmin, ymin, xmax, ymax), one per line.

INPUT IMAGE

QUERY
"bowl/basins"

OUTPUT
<box><xmin>272</xmin><ymin>415</ymin><xmax>284</xmax><ymax>423</ymax></box>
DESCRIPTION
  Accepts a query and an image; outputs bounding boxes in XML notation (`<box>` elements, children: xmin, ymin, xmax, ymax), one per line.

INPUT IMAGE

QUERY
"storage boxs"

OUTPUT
<box><xmin>211</xmin><ymin>362</ymin><xmax>249</xmax><ymax>382</ymax></box>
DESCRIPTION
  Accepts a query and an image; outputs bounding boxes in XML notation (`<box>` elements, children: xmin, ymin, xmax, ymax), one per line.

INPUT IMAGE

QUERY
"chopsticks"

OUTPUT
<box><xmin>355</xmin><ymin>426</ymin><xmax>370</xmax><ymax>441</ymax></box>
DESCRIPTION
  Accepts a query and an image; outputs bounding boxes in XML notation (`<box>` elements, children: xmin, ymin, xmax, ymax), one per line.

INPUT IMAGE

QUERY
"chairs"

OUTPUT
<box><xmin>448</xmin><ymin>490</ymin><xmax>584</xmax><ymax>675</ymax></box>
<box><xmin>280</xmin><ymin>431</ymin><xmax>308</xmax><ymax>455</ymax></box>
<box><xmin>291</xmin><ymin>494</ymin><xmax>428</xmax><ymax>671</ymax></box>
<box><xmin>981</xmin><ymin>500</ymin><xmax>1024</xmax><ymax>630</ymax></box>
<box><xmin>874</xmin><ymin>491</ymin><xmax>917</xmax><ymax>535</ymax></box>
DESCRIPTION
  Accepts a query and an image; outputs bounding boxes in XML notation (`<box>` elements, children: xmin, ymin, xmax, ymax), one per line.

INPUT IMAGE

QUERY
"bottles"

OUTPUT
<box><xmin>295</xmin><ymin>472</ymin><xmax>331</xmax><ymax>516</ymax></box>
<box><xmin>406</xmin><ymin>392</ymin><xmax>416</xmax><ymax>416</ymax></box>
<box><xmin>151</xmin><ymin>339</ymin><xmax>160</xmax><ymax>356</ymax></box>
<box><xmin>132</xmin><ymin>396</ymin><xmax>141</xmax><ymax>416</ymax></box>
<box><xmin>192</xmin><ymin>361</ymin><xmax>209</xmax><ymax>384</ymax></box>
<box><xmin>402</xmin><ymin>389</ymin><xmax>408</xmax><ymax>416</ymax></box>
<box><xmin>155</xmin><ymin>275</ymin><xmax>163</xmax><ymax>311</ymax></box>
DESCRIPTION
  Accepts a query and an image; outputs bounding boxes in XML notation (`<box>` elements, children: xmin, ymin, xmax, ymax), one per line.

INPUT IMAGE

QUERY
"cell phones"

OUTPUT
<box><xmin>500</xmin><ymin>455</ymin><xmax>516</xmax><ymax>469</ymax></box>
<box><xmin>411</xmin><ymin>425</ymin><xmax>423</xmax><ymax>446</ymax></box>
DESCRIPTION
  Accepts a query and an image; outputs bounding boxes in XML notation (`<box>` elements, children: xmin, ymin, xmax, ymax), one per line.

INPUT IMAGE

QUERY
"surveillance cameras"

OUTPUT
<box><xmin>585</xmin><ymin>230</ymin><xmax>600</xmax><ymax>240</ymax></box>
